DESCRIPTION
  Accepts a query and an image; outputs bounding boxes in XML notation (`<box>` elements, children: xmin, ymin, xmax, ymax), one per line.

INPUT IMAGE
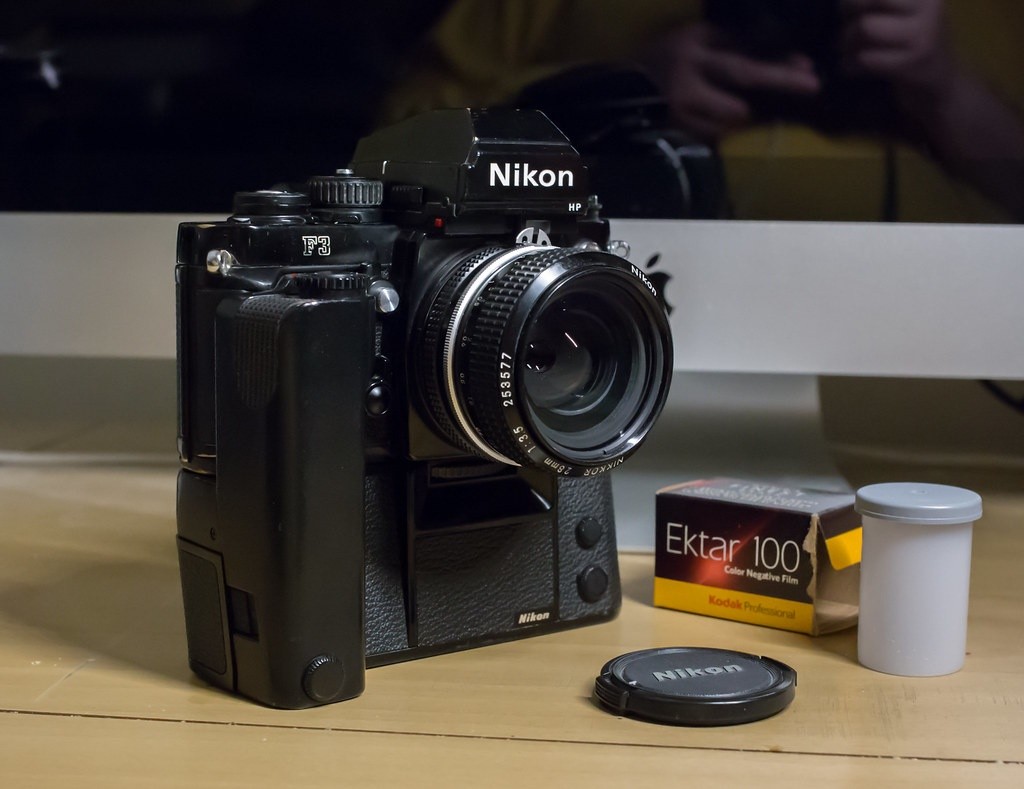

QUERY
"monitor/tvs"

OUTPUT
<box><xmin>0</xmin><ymin>0</ymin><xmax>1024</xmax><ymax>557</ymax></box>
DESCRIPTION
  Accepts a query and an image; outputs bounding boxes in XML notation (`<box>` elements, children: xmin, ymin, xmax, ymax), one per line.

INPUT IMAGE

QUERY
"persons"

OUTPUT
<box><xmin>357</xmin><ymin>0</ymin><xmax>1024</xmax><ymax>226</ymax></box>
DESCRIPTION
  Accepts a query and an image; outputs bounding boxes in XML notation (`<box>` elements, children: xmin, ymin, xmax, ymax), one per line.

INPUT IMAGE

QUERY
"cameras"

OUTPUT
<box><xmin>491</xmin><ymin>67</ymin><xmax>728</xmax><ymax>223</ymax></box>
<box><xmin>169</xmin><ymin>104</ymin><xmax>675</xmax><ymax>711</ymax></box>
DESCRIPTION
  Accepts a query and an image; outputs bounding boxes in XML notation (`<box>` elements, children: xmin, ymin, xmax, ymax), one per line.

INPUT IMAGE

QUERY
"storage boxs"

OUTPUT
<box><xmin>653</xmin><ymin>476</ymin><xmax>863</xmax><ymax>635</ymax></box>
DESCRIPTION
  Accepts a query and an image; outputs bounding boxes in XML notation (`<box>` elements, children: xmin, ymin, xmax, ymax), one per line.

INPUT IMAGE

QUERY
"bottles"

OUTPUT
<box><xmin>855</xmin><ymin>482</ymin><xmax>981</xmax><ymax>677</ymax></box>
